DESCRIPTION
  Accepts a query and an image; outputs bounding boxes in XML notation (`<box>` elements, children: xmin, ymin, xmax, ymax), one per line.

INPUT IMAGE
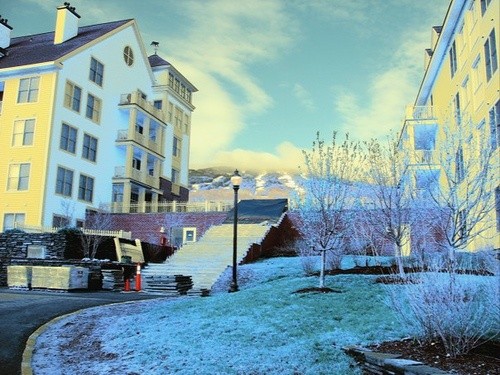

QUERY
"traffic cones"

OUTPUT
<box><xmin>133</xmin><ymin>261</ymin><xmax>142</xmax><ymax>291</ymax></box>
<box><xmin>122</xmin><ymin>278</ymin><xmax>131</xmax><ymax>291</ymax></box>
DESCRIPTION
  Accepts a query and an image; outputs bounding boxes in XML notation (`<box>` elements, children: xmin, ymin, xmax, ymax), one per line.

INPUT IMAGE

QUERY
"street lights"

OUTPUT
<box><xmin>228</xmin><ymin>168</ymin><xmax>242</xmax><ymax>292</ymax></box>
